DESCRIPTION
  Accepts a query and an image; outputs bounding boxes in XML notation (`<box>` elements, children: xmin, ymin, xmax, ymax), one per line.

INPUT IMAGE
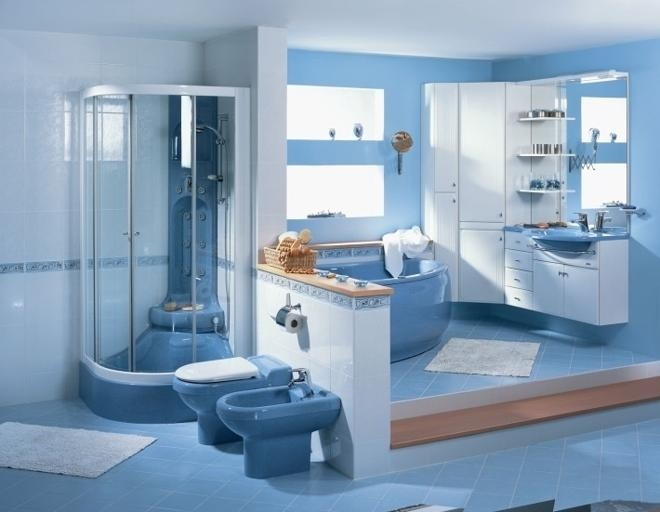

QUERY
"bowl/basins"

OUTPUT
<box><xmin>528</xmin><ymin>110</ymin><xmax>553</xmax><ymax>118</ymax></box>
<box><xmin>317</xmin><ymin>270</ymin><xmax>329</xmax><ymax>277</ymax></box>
<box><xmin>336</xmin><ymin>275</ymin><xmax>349</xmax><ymax>282</ymax></box>
<box><xmin>553</xmin><ymin>111</ymin><xmax>566</xmax><ymax>117</ymax></box>
<box><xmin>354</xmin><ymin>280</ymin><xmax>369</xmax><ymax>287</ymax></box>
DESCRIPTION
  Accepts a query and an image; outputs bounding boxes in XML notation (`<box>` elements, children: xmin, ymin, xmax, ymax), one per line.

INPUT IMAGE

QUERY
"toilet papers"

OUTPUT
<box><xmin>271</xmin><ymin>308</ymin><xmax>302</xmax><ymax>334</ymax></box>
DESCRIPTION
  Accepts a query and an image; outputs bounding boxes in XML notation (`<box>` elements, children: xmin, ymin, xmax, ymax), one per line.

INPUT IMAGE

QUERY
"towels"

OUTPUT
<box><xmin>505</xmin><ymin>229</ymin><xmax>630</xmax><ymax>326</ymax></box>
<box><xmin>382</xmin><ymin>223</ymin><xmax>433</xmax><ymax>279</ymax></box>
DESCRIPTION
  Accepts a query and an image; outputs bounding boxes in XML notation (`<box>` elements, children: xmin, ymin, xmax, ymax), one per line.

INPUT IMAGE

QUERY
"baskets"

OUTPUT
<box><xmin>263</xmin><ymin>246</ymin><xmax>318</xmax><ymax>274</ymax></box>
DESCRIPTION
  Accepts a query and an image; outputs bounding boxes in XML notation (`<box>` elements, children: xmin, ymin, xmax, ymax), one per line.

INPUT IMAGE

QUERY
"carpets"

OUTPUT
<box><xmin>0</xmin><ymin>420</ymin><xmax>159</xmax><ymax>477</ymax></box>
<box><xmin>423</xmin><ymin>337</ymin><xmax>540</xmax><ymax>376</ymax></box>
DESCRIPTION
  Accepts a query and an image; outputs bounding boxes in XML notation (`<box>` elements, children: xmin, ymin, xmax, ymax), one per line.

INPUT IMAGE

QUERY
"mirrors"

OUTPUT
<box><xmin>559</xmin><ymin>70</ymin><xmax>630</xmax><ymax>226</ymax></box>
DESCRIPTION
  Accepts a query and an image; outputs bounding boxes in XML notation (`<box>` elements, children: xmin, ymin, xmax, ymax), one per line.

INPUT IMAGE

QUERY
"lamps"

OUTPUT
<box><xmin>390</xmin><ymin>130</ymin><xmax>414</xmax><ymax>174</ymax></box>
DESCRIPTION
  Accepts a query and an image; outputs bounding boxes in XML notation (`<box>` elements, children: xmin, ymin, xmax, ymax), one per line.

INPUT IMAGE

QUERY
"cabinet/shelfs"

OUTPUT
<box><xmin>419</xmin><ymin>81</ymin><xmax>505</xmax><ymax>305</ymax></box>
<box><xmin>516</xmin><ymin>114</ymin><xmax>562</xmax><ymax>194</ymax></box>
<box><xmin>560</xmin><ymin>116</ymin><xmax>576</xmax><ymax>193</ymax></box>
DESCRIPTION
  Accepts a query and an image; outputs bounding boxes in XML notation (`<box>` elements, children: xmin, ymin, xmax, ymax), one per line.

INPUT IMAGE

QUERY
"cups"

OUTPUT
<box><xmin>543</xmin><ymin>144</ymin><xmax>551</xmax><ymax>154</ymax></box>
<box><xmin>533</xmin><ymin>144</ymin><xmax>542</xmax><ymax>155</ymax></box>
<box><xmin>554</xmin><ymin>144</ymin><xmax>562</xmax><ymax>154</ymax></box>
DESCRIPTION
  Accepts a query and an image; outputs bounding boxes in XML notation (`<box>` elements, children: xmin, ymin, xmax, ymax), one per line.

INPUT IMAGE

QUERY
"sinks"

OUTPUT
<box><xmin>215</xmin><ymin>383</ymin><xmax>343</xmax><ymax>479</ymax></box>
<box><xmin>531</xmin><ymin>233</ymin><xmax>596</xmax><ymax>251</ymax></box>
<box><xmin>593</xmin><ymin>226</ymin><xmax>628</xmax><ymax>235</ymax></box>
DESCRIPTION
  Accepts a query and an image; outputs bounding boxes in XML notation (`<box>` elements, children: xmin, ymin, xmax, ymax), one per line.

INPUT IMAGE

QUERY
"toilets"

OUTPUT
<box><xmin>170</xmin><ymin>353</ymin><xmax>293</xmax><ymax>446</ymax></box>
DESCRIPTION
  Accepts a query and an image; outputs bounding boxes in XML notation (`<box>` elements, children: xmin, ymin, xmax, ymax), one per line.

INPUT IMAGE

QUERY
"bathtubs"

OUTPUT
<box><xmin>309</xmin><ymin>255</ymin><xmax>453</xmax><ymax>366</ymax></box>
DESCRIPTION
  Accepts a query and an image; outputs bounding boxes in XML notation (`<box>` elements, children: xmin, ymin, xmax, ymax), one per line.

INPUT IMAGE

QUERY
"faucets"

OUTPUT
<box><xmin>287</xmin><ymin>366</ymin><xmax>313</xmax><ymax>391</ymax></box>
<box><xmin>570</xmin><ymin>211</ymin><xmax>590</xmax><ymax>233</ymax></box>
<box><xmin>594</xmin><ymin>209</ymin><xmax>612</xmax><ymax>230</ymax></box>
